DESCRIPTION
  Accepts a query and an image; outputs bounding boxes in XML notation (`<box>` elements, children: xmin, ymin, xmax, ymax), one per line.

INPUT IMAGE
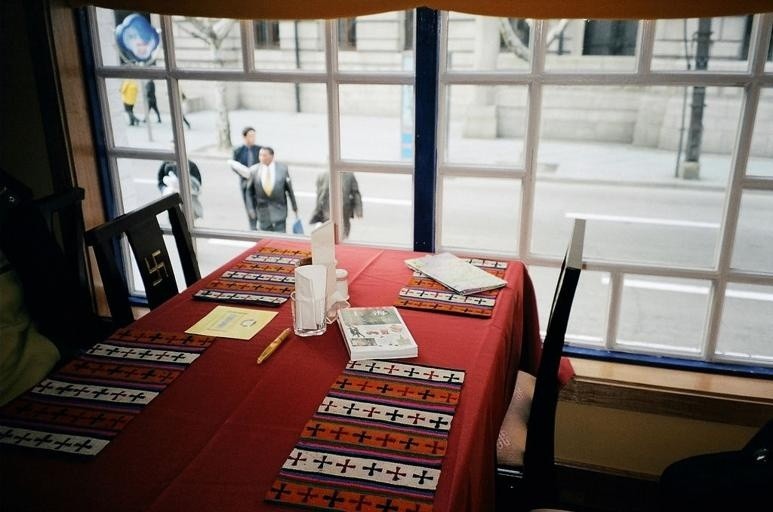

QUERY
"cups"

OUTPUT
<box><xmin>289</xmin><ymin>291</ymin><xmax>327</xmax><ymax>338</ymax></box>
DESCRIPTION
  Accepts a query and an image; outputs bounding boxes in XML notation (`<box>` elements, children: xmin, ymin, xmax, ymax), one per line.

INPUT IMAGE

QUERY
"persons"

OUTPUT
<box><xmin>175</xmin><ymin>78</ymin><xmax>191</xmax><ymax>134</ymax></box>
<box><xmin>230</xmin><ymin>127</ymin><xmax>264</xmax><ymax>230</ymax></box>
<box><xmin>308</xmin><ymin>167</ymin><xmax>364</xmax><ymax>241</ymax></box>
<box><xmin>118</xmin><ymin>79</ymin><xmax>141</xmax><ymax>127</ymax></box>
<box><xmin>155</xmin><ymin>141</ymin><xmax>202</xmax><ymax>229</ymax></box>
<box><xmin>244</xmin><ymin>147</ymin><xmax>300</xmax><ymax>233</ymax></box>
<box><xmin>142</xmin><ymin>79</ymin><xmax>161</xmax><ymax>124</ymax></box>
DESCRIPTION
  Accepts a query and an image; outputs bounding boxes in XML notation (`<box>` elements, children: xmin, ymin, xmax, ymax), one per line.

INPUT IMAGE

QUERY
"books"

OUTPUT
<box><xmin>334</xmin><ymin>306</ymin><xmax>418</xmax><ymax>364</ymax></box>
<box><xmin>401</xmin><ymin>252</ymin><xmax>508</xmax><ymax>296</ymax></box>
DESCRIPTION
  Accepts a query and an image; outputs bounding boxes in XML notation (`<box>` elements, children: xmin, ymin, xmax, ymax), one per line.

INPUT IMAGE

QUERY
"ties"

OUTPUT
<box><xmin>248</xmin><ymin>148</ymin><xmax>253</xmax><ymax>166</ymax></box>
<box><xmin>265</xmin><ymin>166</ymin><xmax>272</xmax><ymax>197</ymax></box>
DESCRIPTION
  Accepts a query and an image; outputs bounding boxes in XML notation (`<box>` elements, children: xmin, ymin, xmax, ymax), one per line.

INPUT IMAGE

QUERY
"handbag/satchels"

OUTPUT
<box><xmin>293</xmin><ymin>220</ymin><xmax>303</xmax><ymax>234</ymax></box>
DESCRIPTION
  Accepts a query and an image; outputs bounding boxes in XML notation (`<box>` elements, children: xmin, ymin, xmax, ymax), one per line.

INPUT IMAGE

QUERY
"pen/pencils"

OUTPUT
<box><xmin>257</xmin><ymin>328</ymin><xmax>292</xmax><ymax>365</ymax></box>
